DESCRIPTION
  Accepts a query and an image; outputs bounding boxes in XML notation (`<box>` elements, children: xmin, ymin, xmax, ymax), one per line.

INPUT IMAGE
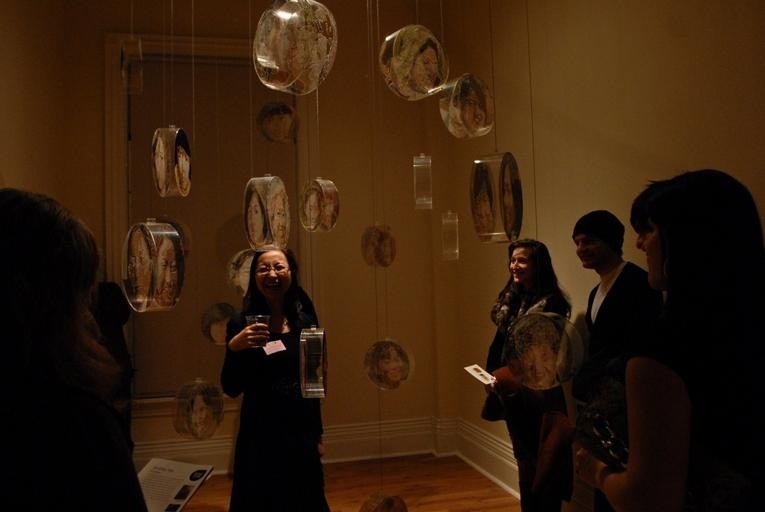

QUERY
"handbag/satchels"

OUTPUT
<box><xmin>578</xmin><ymin>373</ymin><xmax>632</xmax><ymax>465</ymax></box>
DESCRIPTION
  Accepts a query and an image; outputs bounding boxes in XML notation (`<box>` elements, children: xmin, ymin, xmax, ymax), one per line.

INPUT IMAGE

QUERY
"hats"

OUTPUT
<box><xmin>572</xmin><ymin>209</ymin><xmax>625</xmax><ymax>245</ymax></box>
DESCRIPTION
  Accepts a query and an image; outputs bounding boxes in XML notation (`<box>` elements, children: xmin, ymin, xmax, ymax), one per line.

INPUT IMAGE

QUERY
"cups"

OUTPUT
<box><xmin>245</xmin><ymin>315</ymin><xmax>271</xmax><ymax>346</ymax></box>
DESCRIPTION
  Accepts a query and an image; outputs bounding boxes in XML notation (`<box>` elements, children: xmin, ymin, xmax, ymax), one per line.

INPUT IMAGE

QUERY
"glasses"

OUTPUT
<box><xmin>253</xmin><ymin>263</ymin><xmax>292</xmax><ymax>275</ymax></box>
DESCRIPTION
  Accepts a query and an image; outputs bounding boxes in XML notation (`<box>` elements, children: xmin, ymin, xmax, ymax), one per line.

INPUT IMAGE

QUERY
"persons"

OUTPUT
<box><xmin>454</xmin><ymin>75</ymin><xmax>489</xmax><ymax>136</ymax></box>
<box><xmin>231</xmin><ymin>250</ymin><xmax>258</xmax><ymax>297</ymax></box>
<box><xmin>171</xmin><ymin>127</ymin><xmax>193</xmax><ymax>198</ymax></box>
<box><xmin>481</xmin><ymin>238</ymin><xmax>576</xmax><ymax>511</ymax></box>
<box><xmin>575</xmin><ymin>168</ymin><xmax>765</xmax><ymax>512</ymax></box>
<box><xmin>123</xmin><ymin>223</ymin><xmax>158</xmax><ymax>311</ymax></box>
<box><xmin>513</xmin><ymin>313</ymin><xmax>561</xmax><ymax>390</ymax></box>
<box><xmin>361</xmin><ymin>227</ymin><xmax>399</xmax><ymax>268</ymax></box>
<box><xmin>572</xmin><ymin>210</ymin><xmax>662</xmax><ymax>455</ymax></box>
<box><xmin>245</xmin><ymin>179</ymin><xmax>271</xmax><ymax>251</ymax></box>
<box><xmin>266</xmin><ymin>177</ymin><xmax>289</xmax><ymax>249</ymax></box>
<box><xmin>473</xmin><ymin>166</ymin><xmax>495</xmax><ymax>241</ymax></box>
<box><xmin>1</xmin><ymin>187</ymin><xmax>148</xmax><ymax>510</ymax></box>
<box><xmin>364</xmin><ymin>340</ymin><xmax>413</xmax><ymax>393</ymax></box>
<box><xmin>152</xmin><ymin>129</ymin><xmax>171</xmax><ymax>196</ymax></box>
<box><xmin>188</xmin><ymin>383</ymin><xmax>224</xmax><ymax>438</ymax></box>
<box><xmin>201</xmin><ymin>303</ymin><xmax>236</xmax><ymax>346</ymax></box>
<box><xmin>219</xmin><ymin>245</ymin><xmax>333</xmax><ymax>512</ymax></box>
<box><xmin>497</xmin><ymin>151</ymin><xmax>523</xmax><ymax>243</ymax></box>
<box><xmin>152</xmin><ymin>228</ymin><xmax>185</xmax><ymax>309</ymax></box>
<box><xmin>381</xmin><ymin>32</ymin><xmax>441</xmax><ymax>100</ymax></box>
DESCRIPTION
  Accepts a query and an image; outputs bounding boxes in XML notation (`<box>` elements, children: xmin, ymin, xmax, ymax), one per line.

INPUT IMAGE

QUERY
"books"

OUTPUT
<box><xmin>136</xmin><ymin>457</ymin><xmax>214</xmax><ymax>512</ymax></box>
